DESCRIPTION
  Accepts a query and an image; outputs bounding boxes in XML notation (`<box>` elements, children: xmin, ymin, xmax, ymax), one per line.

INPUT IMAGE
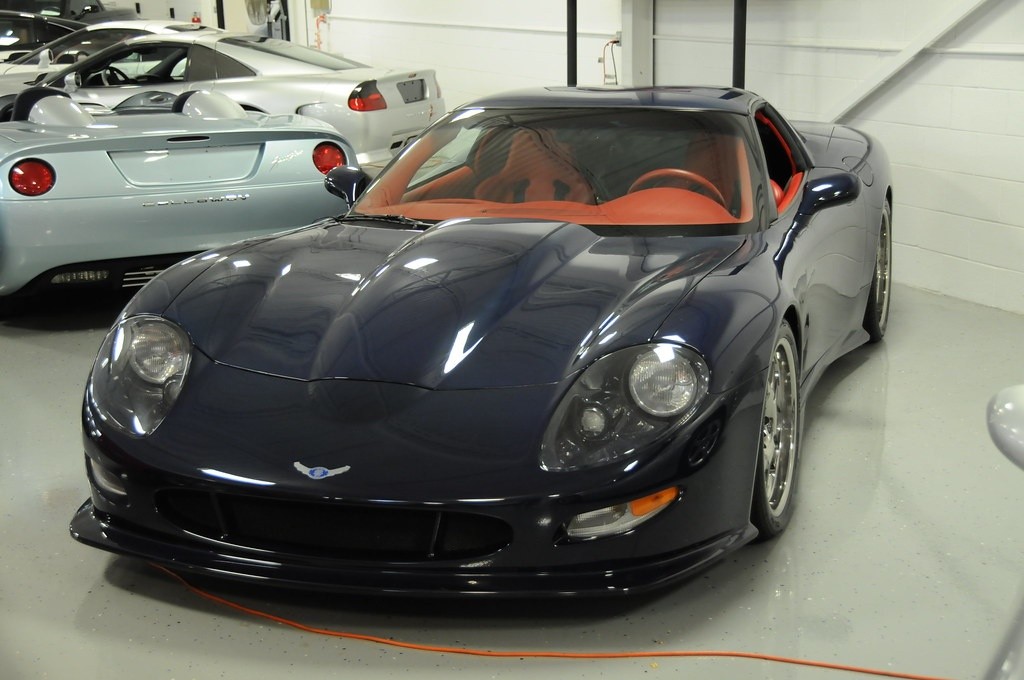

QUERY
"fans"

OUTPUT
<box><xmin>246</xmin><ymin>0</ymin><xmax>287</xmax><ymax>25</ymax></box>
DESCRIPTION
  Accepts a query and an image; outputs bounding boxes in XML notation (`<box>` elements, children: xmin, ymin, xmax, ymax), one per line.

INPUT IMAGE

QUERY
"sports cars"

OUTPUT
<box><xmin>70</xmin><ymin>86</ymin><xmax>894</xmax><ymax>598</ymax></box>
<box><xmin>0</xmin><ymin>87</ymin><xmax>361</xmax><ymax>322</ymax></box>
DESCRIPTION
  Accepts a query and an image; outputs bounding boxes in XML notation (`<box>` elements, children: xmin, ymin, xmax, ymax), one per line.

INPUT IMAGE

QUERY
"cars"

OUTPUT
<box><xmin>0</xmin><ymin>0</ymin><xmax>447</xmax><ymax>187</ymax></box>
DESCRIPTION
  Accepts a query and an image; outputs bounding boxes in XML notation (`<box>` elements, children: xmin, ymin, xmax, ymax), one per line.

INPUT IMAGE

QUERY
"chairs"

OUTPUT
<box><xmin>469</xmin><ymin>128</ymin><xmax>610</xmax><ymax>206</ymax></box>
<box><xmin>655</xmin><ymin>132</ymin><xmax>784</xmax><ymax>212</ymax></box>
